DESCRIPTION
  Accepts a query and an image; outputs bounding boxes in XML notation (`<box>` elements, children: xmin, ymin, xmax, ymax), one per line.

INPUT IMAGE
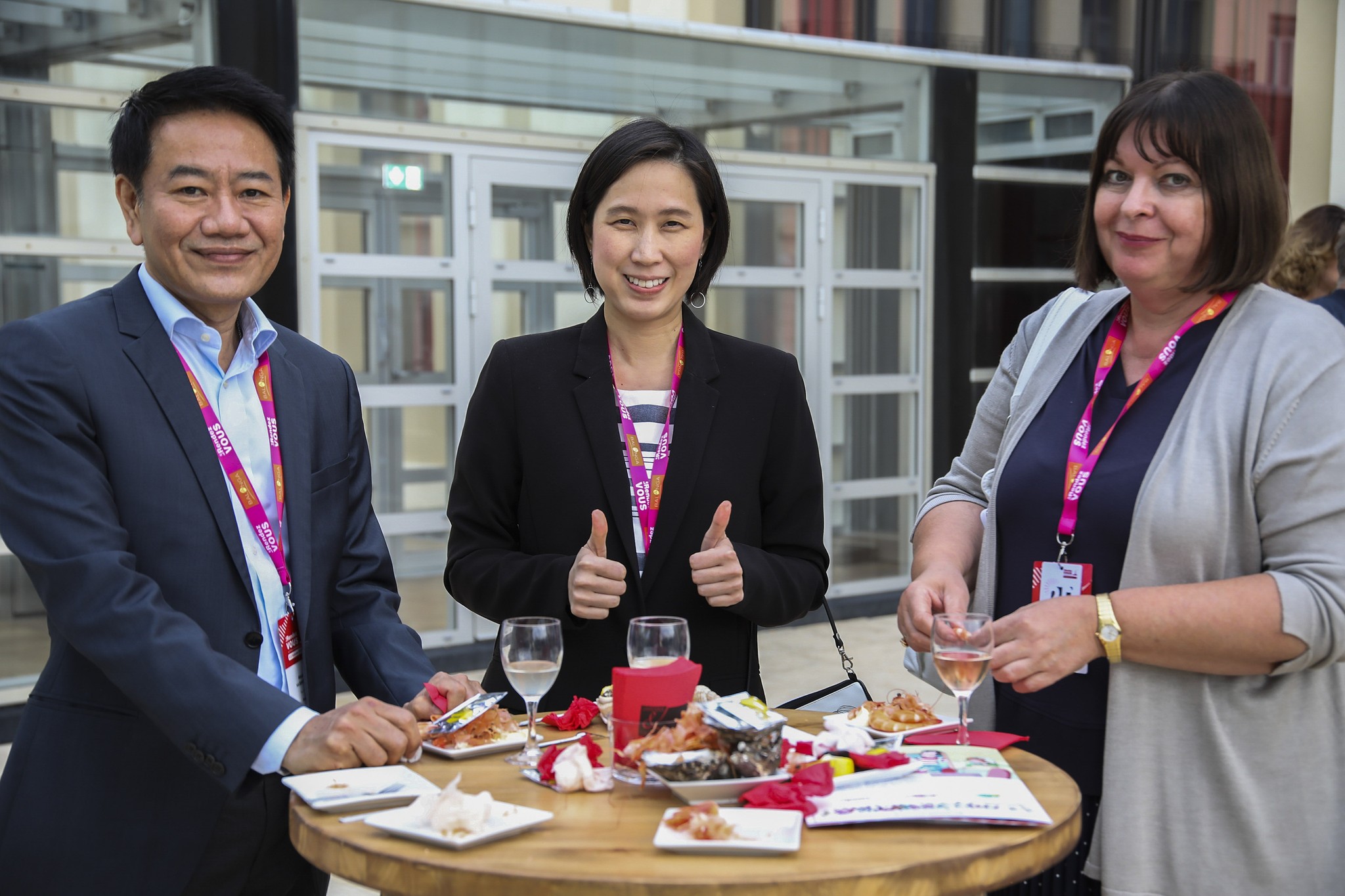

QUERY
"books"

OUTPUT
<box><xmin>800</xmin><ymin>745</ymin><xmax>1055</xmax><ymax>830</ymax></box>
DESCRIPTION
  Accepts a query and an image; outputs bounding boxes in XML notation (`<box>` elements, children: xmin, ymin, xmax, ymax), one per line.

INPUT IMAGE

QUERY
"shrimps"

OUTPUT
<box><xmin>847</xmin><ymin>688</ymin><xmax>943</xmax><ymax>733</ymax></box>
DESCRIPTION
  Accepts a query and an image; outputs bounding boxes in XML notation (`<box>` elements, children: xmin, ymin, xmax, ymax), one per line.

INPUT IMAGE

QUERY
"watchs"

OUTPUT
<box><xmin>1093</xmin><ymin>592</ymin><xmax>1124</xmax><ymax>665</ymax></box>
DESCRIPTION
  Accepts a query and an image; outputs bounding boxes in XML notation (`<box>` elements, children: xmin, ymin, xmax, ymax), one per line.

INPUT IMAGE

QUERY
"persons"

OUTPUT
<box><xmin>1301</xmin><ymin>222</ymin><xmax>1345</xmax><ymax>324</ymax></box>
<box><xmin>440</xmin><ymin>116</ymin><xmax>833</xmax><ymax>706</ymax></box>
<box><xmin>897</xmin><ymin>64</ymin><xmax>1345</xmax><ymax>896</ymax></box>
<box><xmin>1262</xmin><ymin>203</ymin><xmax>1345</xmax><ymax>301</ymax></box>
<box><xmin>1</xmin><ymin>61</ymin><xmax>493</xmax><ymax>896</ymax></box>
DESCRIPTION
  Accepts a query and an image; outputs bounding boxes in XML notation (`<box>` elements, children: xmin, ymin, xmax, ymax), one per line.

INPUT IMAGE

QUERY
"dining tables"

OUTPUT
<box><xmin>287</xmin><ymin>708</ymin><xmax>1082</xmax><ymax>896</ymax></box>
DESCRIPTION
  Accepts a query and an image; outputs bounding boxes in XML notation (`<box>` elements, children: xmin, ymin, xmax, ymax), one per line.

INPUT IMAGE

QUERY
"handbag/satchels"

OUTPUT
<box><xmin>774</xmin><ymin>679</ymin><xmax>873</xmax><ymax>713</ymax></box>
<box><xmin>903</xmin><ymin>465</ymin><xmax>998</xmax><ymax>697</ymax></box>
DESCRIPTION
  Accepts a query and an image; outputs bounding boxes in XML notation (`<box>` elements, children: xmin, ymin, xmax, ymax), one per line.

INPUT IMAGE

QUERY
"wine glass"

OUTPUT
<box><xmin>930</xmin><ymin>614</ymin><xmax>992</xmax><ymax>745</ymax></box>
<box><xmin>498</xmin><ymin>616</ymin><xmax>563</xmax><ymax>766</ymax></box>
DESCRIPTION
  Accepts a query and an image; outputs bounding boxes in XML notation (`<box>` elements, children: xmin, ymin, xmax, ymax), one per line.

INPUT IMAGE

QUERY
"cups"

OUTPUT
<box><xmin>627</xmin><ymin>615</ymin><xmax>690</xmax><ymax>671</ymax></box>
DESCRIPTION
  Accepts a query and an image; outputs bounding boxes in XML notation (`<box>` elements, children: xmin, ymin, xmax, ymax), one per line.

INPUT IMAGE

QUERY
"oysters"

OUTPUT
<box><xmin>642</xmin><ymin>722</ymin><xmax>782</xmax><ymax>781</ymax></box>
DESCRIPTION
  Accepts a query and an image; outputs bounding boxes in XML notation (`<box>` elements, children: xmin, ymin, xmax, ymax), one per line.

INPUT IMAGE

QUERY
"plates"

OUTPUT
<box><xmin>280</xmin><ymin>686</ymin><xmax>970</xmax><ymax>855</ymax></box>
